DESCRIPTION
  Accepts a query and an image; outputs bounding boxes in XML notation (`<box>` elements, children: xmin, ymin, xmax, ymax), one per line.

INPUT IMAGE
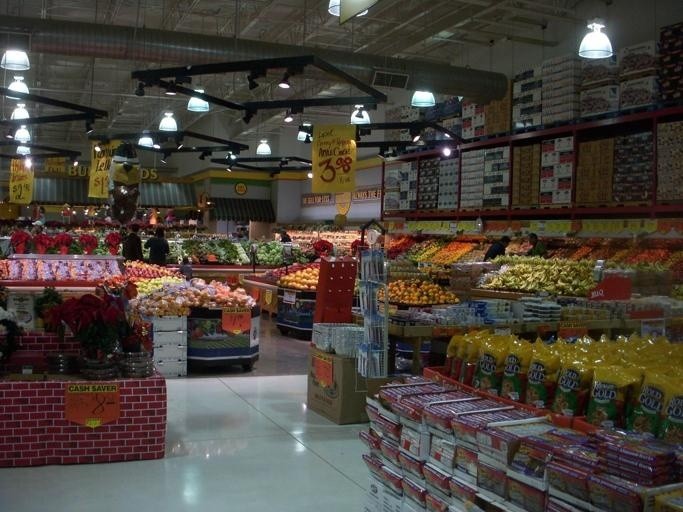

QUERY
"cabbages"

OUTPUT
<box><xmin>256</xmin><ymin>241</ymin><xmax>284</xmax><ymax>266</ymax></box>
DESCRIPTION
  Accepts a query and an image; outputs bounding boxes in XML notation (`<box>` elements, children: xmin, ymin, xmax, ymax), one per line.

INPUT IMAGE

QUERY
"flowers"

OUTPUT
<box><xmin>99</xmin><ymin>279</ymin><xmax>153</xmax><ymax>352</ymax></box>
<box><xmin>58</xmin><ymin>295</ymin><xmax>117</xmax><ymax>358</ymax></box>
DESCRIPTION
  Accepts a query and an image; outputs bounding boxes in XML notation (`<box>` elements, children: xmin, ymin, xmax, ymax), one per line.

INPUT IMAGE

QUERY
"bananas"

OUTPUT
<box><xmin>480</xmin><ymin>254</ymin><xmax>683</xmax><ymax>296</ymax></box>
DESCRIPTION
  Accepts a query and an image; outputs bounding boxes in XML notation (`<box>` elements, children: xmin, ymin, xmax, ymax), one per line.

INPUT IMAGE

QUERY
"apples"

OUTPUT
<box><xmin>378</xmin><ymin>279</ymin><xmax>462</xmax><ymax>305</ymax></box>
<box><xmin>267</xmin><ymin>263</ymin><xmax>321</xmax><ymax>290</ymax></box>
<box><xmin>125</xmin><ymin>260</ymin><xmax>256</xmax><ymax>316</ymax></box>
<box><xmin>627</xmin><ymin>248</ymin><xmax>670</xmax><ymax>263</ymax></box>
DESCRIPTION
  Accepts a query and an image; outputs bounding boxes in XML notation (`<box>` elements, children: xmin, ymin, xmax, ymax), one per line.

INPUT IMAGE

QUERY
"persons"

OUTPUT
<box><xmin>123</xmin><ymin>223</ymin><xmax>143</xmax><ymax>260</ymax></box>
<box><xmin>525</xmin><ymin>232</ymin><xmax>546</xmax><ymax>257</ymax></box>
<box><xmin>281</xmin><ymin>231</ymin><xmax>291</xmax><ymax>242</ymax></box>
<box><xmin>179</xmin><ymin>257</ymin><xmax>193</xmax><ymax>281</ymax></box>
<box><xmin>144</xmin><ymin>226</ymin><xmax>170</xmax><ymax>264</ymax></box>
<box><xmin>483</xmin><ymin>235</ymin><xmax>511</xmax><ymax>262</ymax></box>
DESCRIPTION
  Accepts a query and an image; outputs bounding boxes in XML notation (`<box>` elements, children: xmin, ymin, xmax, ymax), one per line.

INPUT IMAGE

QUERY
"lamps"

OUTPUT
<box><xmin>411</xmin><ymin>90</ymin><xmax>435</xmax><ymax>107</ymax></box>
<box><xmin>5</xmin><ymin>75</ymin><xmax>28</xmax><ymax>100</ymax></box>
<box><xmin>186</xmin><ymin>74</ymin><xmax>209</xmax><ymax>112</ymax></box>
<box><xmin>350</xmin><ymin>104</ymin><xmax>370</xmax><ymax>124</ymax></box>
<box><xmin>255</xmin><ymin>137</ymin><xmax>270</xmax><ymax>155</ymax></box>
<box><xmin>13</xmin><ymin>124</ymin><xmax>30</xmax><ymax>141</ymax></box>
<box><xmin>296</xmin><ymin>122</ymin><xmax>313</xmax><ymax>143</ymax></box>
<box><xmin>137</xmin><ymin>130</ymin><xmax>153</xmax><ymax>147</ymax></box>
<box><xmin>9</xmin><ymin>103</ymin><xmax>29</xmax><ymax>120</ymax></box>
<box><xmin>576</xmin><ymin>17</ymin><xmax>612</xmax><ymax>59</ymax></box>
<box><xmin>158</xmin><ymin>112</ymin><xmax>177</xmax><ymax>132</ymax></box>
<box><xmin>0</xmin><ymin>49</ymin><xmax>30</xmax><ymax>70</ymax></box>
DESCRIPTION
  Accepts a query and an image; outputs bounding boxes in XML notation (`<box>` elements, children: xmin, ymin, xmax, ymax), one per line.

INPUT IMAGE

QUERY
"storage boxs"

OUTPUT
<box><xmin>306</xmin><ymin>346</ymin><xmax>386</xmax><ymax>426</ymax></box>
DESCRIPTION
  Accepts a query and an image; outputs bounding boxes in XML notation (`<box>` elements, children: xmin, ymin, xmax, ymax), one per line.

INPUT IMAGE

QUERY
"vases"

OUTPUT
<box><xmin>43</xmin><ymin>302</ymin><xmax>66</xmax><ymax>350</ymax></box>
<box><xmin>119</xmin><ymin>353</ymin><xmax>152</xmax><ymax>378</ymax></box>
<box><xmin>45</xmin><ymin>352</ymin><xmax>78</xmax><ymax>374</ymax></box>
<box><xmin>77</xmin><ymin>353</ymin><xmax>116</xmax><ymax>379</ymax></box>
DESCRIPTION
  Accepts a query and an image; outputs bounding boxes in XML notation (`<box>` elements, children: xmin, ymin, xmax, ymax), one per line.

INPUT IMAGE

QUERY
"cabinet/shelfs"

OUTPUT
<box><xmin>380</xmin><ymin>105</ymin><xmax>682</xmax><ymax>219</ymax></box>
